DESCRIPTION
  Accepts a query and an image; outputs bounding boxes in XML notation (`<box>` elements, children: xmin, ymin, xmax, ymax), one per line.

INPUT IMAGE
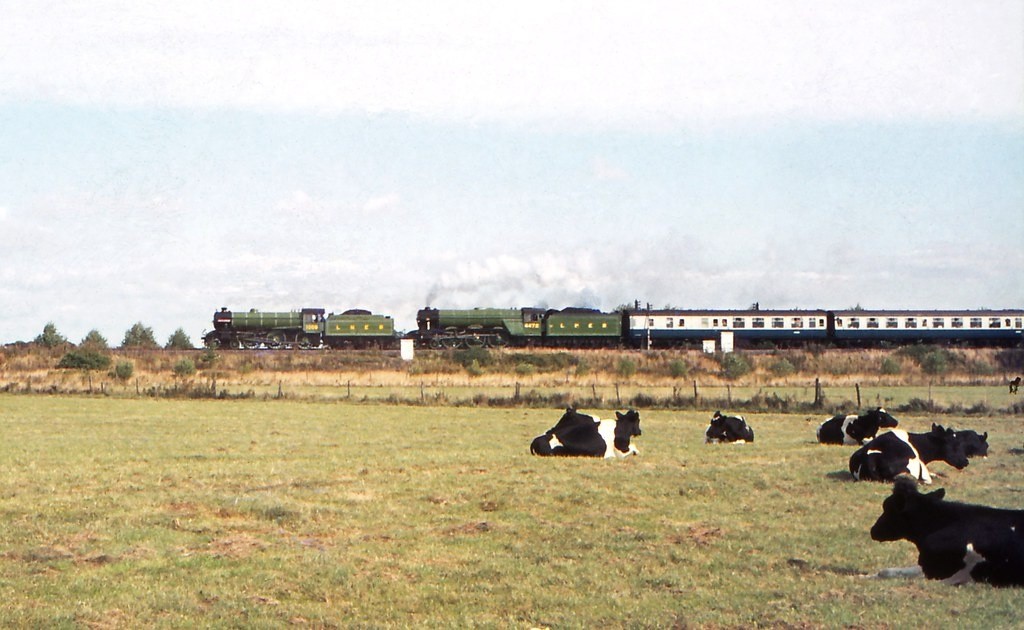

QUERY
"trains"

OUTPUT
<box><xmin>199</xmin><ymin>306</ymin><xmax>1023</xmax><ymax>348</ymax></box>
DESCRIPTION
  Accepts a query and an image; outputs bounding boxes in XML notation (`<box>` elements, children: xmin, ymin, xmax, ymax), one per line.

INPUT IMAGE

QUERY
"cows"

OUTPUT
<box><xmin>870</xmin><ymin>474</ymin><xmax>1024</xmax><ymax>589</ymax></box>
<box><xmin>530</xmin><ymin>405</ymin><xmax>642</xmax><ymax>459</ymax></box>
<box><xmin>704</xmin><ymin>411</ymin><xmax>754</xmax><ymax>446</ymax></box>
<box><xmin>848</xmin><ymin>422</ymin><xmax>989</xmax><ymax>485</ymax></box>
<box><xmin>1009</xmin><ymin>377</ymin><xmax>1022</xmax><ymax>394</ymax></box>
<box><xmin>816</xmin><ymin>405</ymin><xmax>898</xmax><ymax>446</ymax></box>
